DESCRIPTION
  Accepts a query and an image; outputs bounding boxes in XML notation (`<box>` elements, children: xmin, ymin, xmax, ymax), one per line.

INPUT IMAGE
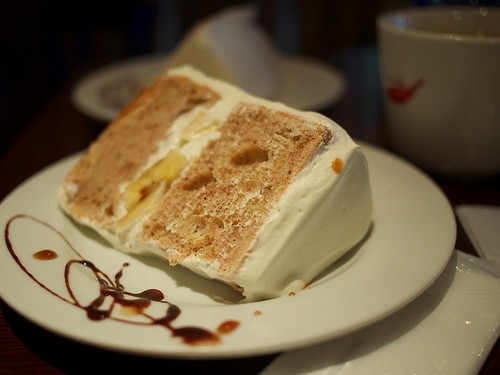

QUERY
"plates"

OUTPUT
<box><xmin>75</xmin><ymin>56</ymin><xmax>345</xmax><ymax>131</ymax></box>
<box><xmin>0</xmin><ymin>138</ymin><xmax>458</xmax><ymax>356</ymax></box>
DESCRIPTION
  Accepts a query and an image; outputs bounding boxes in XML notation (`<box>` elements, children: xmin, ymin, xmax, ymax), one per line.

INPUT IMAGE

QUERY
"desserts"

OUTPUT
<box><xmin>56</xmin><ymin>65</ymin><xmax>373</xmax><ymax>302</ymax></box>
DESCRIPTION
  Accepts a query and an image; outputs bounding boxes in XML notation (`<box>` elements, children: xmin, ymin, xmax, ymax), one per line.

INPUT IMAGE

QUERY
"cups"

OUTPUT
<box><xmin>375</xmin><ymin>8</ymin><xmax>500</xmax><ymax>167</ymax></box>
